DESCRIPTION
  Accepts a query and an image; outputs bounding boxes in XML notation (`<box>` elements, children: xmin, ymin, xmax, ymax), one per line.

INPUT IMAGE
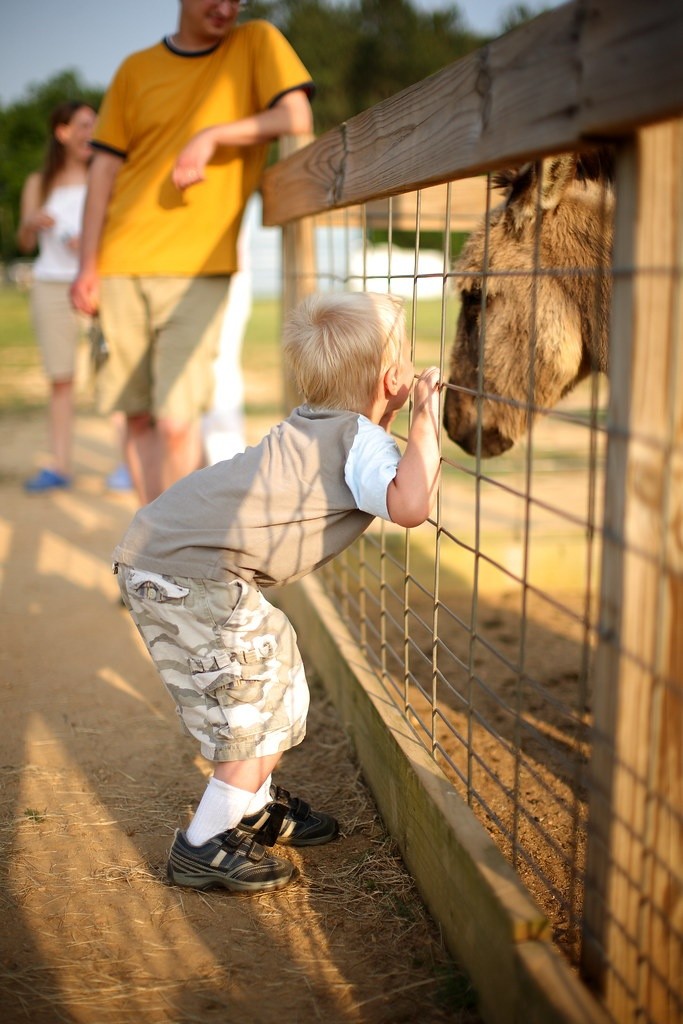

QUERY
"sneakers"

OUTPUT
<box><xmin>166</xmin><ymin>828</ymin><xmax>296</xmax><ymax>893</ymax></box>
<box><xmin>238</xmin><ymin>783</ymin><xmax>340</xmax><ymax>847</ymax></box>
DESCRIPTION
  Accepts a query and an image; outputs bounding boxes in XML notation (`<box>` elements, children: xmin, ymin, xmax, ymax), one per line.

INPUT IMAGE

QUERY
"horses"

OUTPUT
<box><xmin>439</xmin><ymin>148</ymin><xmax>614</xmax><ymax>462</ymax></box>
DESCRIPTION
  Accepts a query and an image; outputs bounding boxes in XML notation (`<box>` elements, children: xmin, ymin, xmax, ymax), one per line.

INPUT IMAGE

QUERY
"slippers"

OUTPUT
<box><xmin>25</xmin><ymin>470</ymin><xmax>68</xmax><ymax>489</ymax></box>
<box><xmin>109</xmin><ymin>469</ymin><xmax>132</xmax><ymax>488</ymax></box>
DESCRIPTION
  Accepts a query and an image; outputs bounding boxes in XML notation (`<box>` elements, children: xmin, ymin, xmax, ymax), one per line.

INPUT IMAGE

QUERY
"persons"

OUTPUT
<box><xmin>68</xmin><ymin>0</ymin><xmax>313</xmax><ymax>607</ymax></box>
<box><xmin>19</xmin><ymin>104</ymin><xmax>133</xmax><ymax>491</ymax></box>
<box><xmin>112</xmin><ymin>293</ymin><xmax>446</xmax><ymax>889</ymax></box>
